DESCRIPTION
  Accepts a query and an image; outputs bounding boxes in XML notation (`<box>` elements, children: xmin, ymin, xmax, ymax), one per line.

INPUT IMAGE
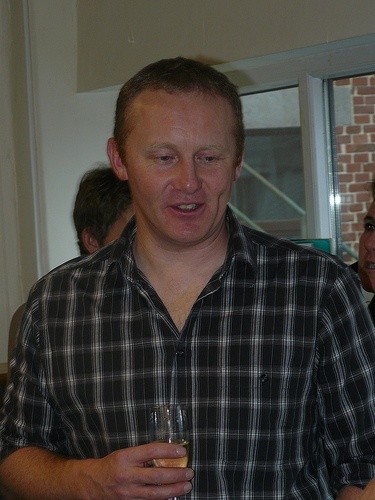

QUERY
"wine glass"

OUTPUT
<box><xmin>154</xmin><ymin>403</ymin><xmax>189</xmax><ymax>500</ymax></box>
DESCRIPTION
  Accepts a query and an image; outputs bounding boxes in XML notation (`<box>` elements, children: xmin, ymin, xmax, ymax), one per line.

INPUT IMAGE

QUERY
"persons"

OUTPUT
<box><xmin>0</xmin><ymin>56</ymin><xmax>375</xmax><ymax>500</ymax></box>
<box><xmin>73</xmin><ymin>166</ymin><xmax>133</xmax><ymax>257</ymax></box>
<box><xmin>358</xmin><ymin>178</ymin><xmax>375</xmax><ymax>328</ymax></box>
